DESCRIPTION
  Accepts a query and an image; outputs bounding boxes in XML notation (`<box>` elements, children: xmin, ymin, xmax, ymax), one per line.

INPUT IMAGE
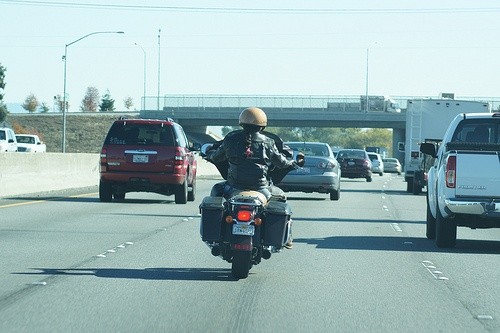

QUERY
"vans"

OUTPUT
<box><xmin>0</xmin><ymin>127</ymin><xmax>18</xmax><ymax>152</ymax></box>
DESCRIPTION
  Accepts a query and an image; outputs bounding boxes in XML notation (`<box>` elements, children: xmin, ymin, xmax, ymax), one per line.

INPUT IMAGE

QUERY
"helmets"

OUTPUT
<box><xmin>239</xmin><ymin>107</ymin><xmax>268</xmax><ymax>127</ymax></box>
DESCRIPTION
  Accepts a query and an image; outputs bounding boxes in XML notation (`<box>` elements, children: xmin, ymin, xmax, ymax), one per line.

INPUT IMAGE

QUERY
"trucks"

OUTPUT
<box><xmin>397</xmin><ymin>97</ymin><xmax>491</xmax><ymax>196</ymax></box>
<box><xmin>326</xmin><ymin>95</ymin><xmax>402</xmax><ymax>114</ymax></box>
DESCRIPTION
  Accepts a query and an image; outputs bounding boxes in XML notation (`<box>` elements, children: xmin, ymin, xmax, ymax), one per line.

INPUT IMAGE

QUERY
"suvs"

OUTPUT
<box><xmin>98</xmin><ymin>117</ymin><xmax>202</xmax><ymax>204</ymax></box>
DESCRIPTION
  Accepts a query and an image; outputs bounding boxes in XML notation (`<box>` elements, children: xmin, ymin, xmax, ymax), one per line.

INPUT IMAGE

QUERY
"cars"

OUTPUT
<box><xmin>367</xmin><ymin>152</ymin><xmax>385</xmax><ymax>176</ymax></box>
<box><xmin>336</xmin><ymin>149</ymin><xmax>374</xmax><ymax>182</ymax></box>
<box><xmin>15</xmin><ymin>134</ymin><xmax>47</xmax><ymax>152</ymax></box>
<box><xmin>383</xmin><ymin>158</ymin><xmax>404</xmax><ymax>175</ymax></box>
<box><xmin>273</xmin><ymin>140</ymin><xmax>345</xmax><ymax>201</ymax></box>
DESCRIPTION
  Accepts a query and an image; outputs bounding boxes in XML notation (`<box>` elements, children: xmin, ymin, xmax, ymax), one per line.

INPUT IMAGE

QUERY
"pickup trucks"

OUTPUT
<box><xmin>418</xmin><ymin>112</ymin><xmax>500</xmax><ymax>250</ymax></box>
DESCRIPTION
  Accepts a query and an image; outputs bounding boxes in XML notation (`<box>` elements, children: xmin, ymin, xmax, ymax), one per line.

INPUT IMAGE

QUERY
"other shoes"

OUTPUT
<box><xmin>282</xmin><ymin>241</ymin><xmax>293</xmax><ymax>249</ymax></box>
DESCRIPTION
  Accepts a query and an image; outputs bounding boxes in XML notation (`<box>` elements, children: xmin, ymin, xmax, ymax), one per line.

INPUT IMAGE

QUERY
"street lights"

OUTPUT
<box><xmin>62</xmin><ymin>31</ymin><xmax>124</xmax><ymax>154</ymax></box>
<box><xmin>134</xmin><ymin>42</ymin><xmax>146</xmax><ymax>111</ymax></box>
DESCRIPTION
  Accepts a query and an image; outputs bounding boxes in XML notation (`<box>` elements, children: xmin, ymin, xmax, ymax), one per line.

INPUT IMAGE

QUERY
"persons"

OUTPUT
<box><xmin>202</xmin><ymin>107</ymin><xmax>297</xmax><ymax>250</ymax></box>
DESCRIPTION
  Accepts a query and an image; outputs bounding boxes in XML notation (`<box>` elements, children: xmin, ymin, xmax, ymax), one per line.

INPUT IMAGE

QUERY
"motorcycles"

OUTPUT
<box><xmin>196</xmin><ymin>132</ymin><xmax>306</xmax><ymax>279</ymax></box>
